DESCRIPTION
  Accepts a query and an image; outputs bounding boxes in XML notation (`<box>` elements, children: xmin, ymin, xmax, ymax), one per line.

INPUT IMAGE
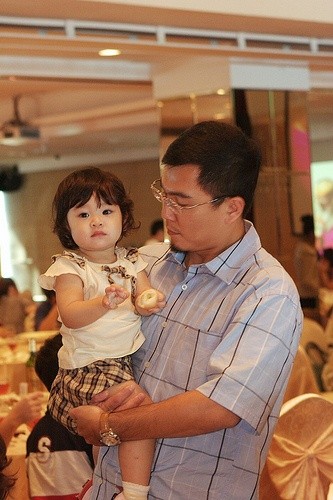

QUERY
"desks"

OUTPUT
<box><xmin>0</xmin><ymin>331</ymin><xmax>54</xmax><ymax>500</ymax></box>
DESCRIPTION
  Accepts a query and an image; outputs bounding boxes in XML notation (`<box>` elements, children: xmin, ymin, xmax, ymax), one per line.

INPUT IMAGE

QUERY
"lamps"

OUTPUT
<box><xmin>0</xmin><ymin>98</ymin><xmax>40</xmax><ymax>145</ymax></box>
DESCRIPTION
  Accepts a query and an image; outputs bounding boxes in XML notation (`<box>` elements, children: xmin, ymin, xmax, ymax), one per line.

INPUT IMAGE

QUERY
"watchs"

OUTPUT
<box><xmin>99</xmin><ymin>411</ymin><xmax>122</xmax><ymax>446</ymax></box>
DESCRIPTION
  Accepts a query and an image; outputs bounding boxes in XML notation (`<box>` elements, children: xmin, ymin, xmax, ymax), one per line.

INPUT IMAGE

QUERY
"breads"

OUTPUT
<box><xmin>135</xmin><ymin>290</ymin><xmax>157</xmax><ymax>309</ymax></box>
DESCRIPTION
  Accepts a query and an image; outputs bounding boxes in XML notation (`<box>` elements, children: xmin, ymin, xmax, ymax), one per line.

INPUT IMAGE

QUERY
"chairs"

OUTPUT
<box><xmin>266</xmin><ymin>316</ymin><xmax>333</xmax><ymax>500</ymax></box>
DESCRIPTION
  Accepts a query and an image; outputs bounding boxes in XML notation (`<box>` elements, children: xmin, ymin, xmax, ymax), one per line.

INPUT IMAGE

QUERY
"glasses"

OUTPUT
<box><xmin>149</xmin><ymin>178</ymin><xmax>229</xmax><ymax>216</ymax></box>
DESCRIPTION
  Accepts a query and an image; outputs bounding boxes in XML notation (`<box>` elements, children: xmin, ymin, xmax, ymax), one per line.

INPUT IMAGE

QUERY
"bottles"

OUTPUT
<box><xmin>24</xmin><ymin>339</ymin><xmax>38</xmax><ymax>367</ymax></box>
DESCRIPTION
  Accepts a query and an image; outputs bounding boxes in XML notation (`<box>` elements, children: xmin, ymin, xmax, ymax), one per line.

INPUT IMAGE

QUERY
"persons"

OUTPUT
<box><xmin>144</xmin><ymin>219</ymin><xmax>164</xmax><ymax>245</ymax></box>
<box><xmin>0</xmin><ymin>277</ymin><xmax>96</xmax><ymax>500</ymax></box>
<box><xmin>294</xmin><ymin>214</ymin><xmax>333</xmax><ymax>392</ymax></box>
<box><xmin>37</xmin><ymin>166</ymin><xmax>167</xmax><ymax>500</ymax></box>
<box><xmin>68</xmin><ymin>120</ymin><xmax>303</xmax><ymax>500</ymax></box>
